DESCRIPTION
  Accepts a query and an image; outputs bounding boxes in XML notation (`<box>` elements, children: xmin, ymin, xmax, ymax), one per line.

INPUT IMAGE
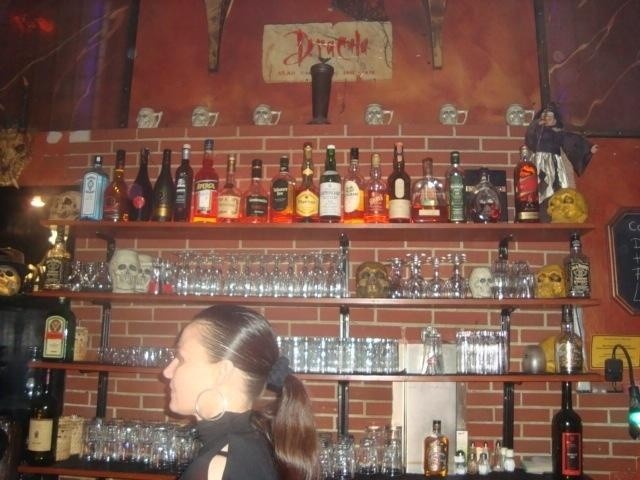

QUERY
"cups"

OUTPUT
<box><xmin>57</xmin><ymin>415</ymin><xmax>200</xmax><ymax>472</ymax></box>
<box><xmin>96</xmin><ymin>344</ymin><xmax>173</xmax><ymax>368</ymax></box>
<box><xmin>73</xmin><ymin>258</ymin><xmax>112</xmax><ymax>291</ymax></box>
<box><xmin>455</xmin><ymin>330</ymin><xmax>510</xmax><ymax>376</ymax></box>
<box><xmin>365</xmin><ymin>102</ymin><xmax>394</xmax><ymax>127</ymax></box>
<box><xmin>22</xmin><ymin>344</ymin><xmax>41</xmax><ymax>398</ymax></box>
<box><xmin>492</xmin><ymin>257</ymin><xmax>537</xmax><ymax>299</ymax></box>
<box><xmin>73</xmin><ymin>326</ymin><xmax>91</xmax><ymax>362</ymax></box>
<box><xmin>421</xmin><ymin>328</ymin><xmax>444</xmax><ymax>374</ymax></box>
<box><xmin>436</xmin><ymin>101</ymin><xmax>465</xmax><ymax>124</ymax></box>
<box><xmin>274</xmin><ymin>336</ymin><xmax>405</xmax><ymax>377</ymax></box>
<box><xmin>504</xmin><ymin>103</ymin><xmax>536</xmax><ymax>128</ymax></box>
<box><xmin>151</xmin><ymin>258</ymin><xmax>178</xmax><ymax>294</ymax></box>
<box><xmin>129</xmin><ymin>106</ymin><xmax>282</xmax><ymax>129</ymax></box>
<box><xmin>318</xmin><ymin>426</ymin><xmax>404</xmax><ymax>480</ymax></box>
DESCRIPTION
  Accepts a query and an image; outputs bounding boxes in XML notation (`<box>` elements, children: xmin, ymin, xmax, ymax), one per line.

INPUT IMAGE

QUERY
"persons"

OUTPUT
<box><xmin>161</xmin><ymin>304</ymin><xmax>322</xmax><ymax>480</ymax></box>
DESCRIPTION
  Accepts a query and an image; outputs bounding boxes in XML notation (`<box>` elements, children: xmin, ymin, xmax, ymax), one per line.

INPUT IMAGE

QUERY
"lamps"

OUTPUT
<box><xmin>604</xmin><ymin>340</ymin><xmax>639</xmax><ymax>442</ymax></box>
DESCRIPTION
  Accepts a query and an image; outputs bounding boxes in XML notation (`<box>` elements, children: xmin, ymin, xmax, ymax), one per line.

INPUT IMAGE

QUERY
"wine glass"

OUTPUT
<box><xmin>390</xmin><ymin>250</ymin><xmax>469</xmax><ymax>300</ymax></box>
<box><xmin>178</xmin><ymin>246</ymin><xmax>347</xmax><ymax>300</ymax></box>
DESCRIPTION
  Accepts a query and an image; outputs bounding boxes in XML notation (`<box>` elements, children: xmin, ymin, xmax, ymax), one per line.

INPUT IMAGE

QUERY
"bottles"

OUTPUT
<box><xmin>367</xmin><ymin>155</ymin><xmax>387</xmax><ymax>222</ymax></box>
<box><xmin>468</xmin><ymin>170</ymin><xmax>499</xmax><ymax>224</ymax></box>
<box><xmin>320</xmin><ymin>145</ymin><xmax>344</xmax><ymax>222</ymax></box>
<box><xmin>152</xmin><ymin>149</ymin><xmax>175</xmax><ymax>222</ymax></box>
<box><xmin>562</xmin><ymin>230</ymin><xmax>593</xmax><ymax>296</ymax></box>
<box><xmin>422</xmin><ymin>419</ymin><xmax>448</xmax><ymax>478</ymax></box>
<box><xmin>387</xmin><ymin>140</ymin><xmax>414</xmax><ymax>223</ymax></box>
<box><xmin>42</xmin><ymin>296</ymin><xmax>73</xmax><ymax>362</ymax></box>
<box><xmin>83</xmin><ymin>153</ymin><xmax>108</xmax><ymax>225</ymax></box>
<box><xmin>456</xmin><ymin>440</ymin><xmax>516</xmax><ymax>478</ymax></box>
<box><xmin>447</xmin><ymin>150</ymin><xmax>468</xmax><ymax>222</ymax></box>
<box><xmin>552</xmin><ymin>381</ymin><xmax>583</xmax><ymax>478</ymax></box>
<box><xmin>24</xmin><ymin>369</ymin><xmax>56</xmax><ymax>467</ymax></box>
<box><xmin>294</xmin><ymin>142</ymin><xmax>322</xmax><ymax>222</ymax></box>
<box><xmin>190</xmin><ymin>140</ymin><xmax>220</xmax><ymax>224</ymax></box>
<box><xmin>104</xmin><ymin>147</ymin><xmax>128</xmax><ymax>223</ymax></box>
<box><xmin>126</xmin><ymin>149</ymin><xmax>157</xmax><ymax>223</ymax></box>
<box><xmin>412</xmin><ymin>160</ymin><xmax>448</xmax><ymax>223</ymax></box>
<box><xmin>44</xmin><ymin>224</ymin><xmax>73</xmax><ymax>290</ymax></box>
<box><xmin>511</xmin><ymin>145</ymin><xmax>541</xmax><ymax>223</ymax></box>
<box><xmin>554</xmin><ymin>303</ymin><xmax>584</xmax><ymax>374</ymax></box>
<box><xmin>269</xmin><ymin>156</ymin><xmax>299</xmax><ymax>222</ymax></box>
<box><xmin>342</xmin><ymin>147</ymin><xmax>365</xmax><ymax>222</ymax></box>
<box><xmin>218</xmin><ymin>153</ymin><xmax>245</xmax><ymax>221</ymax></box>
<box><xmin>244</xmin><ymin>160</ymin><xmax>273</xmax><ymax>224</ymax></box>
<box><xmin>175</xmin><ymin>142</ymin><xmax>193</xmax><ymax>220</ymax></box>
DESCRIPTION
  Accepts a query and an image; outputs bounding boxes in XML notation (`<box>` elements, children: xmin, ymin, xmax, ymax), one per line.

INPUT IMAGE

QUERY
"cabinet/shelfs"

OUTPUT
<box><xmin>16</xmin><ymin>219</ymin><xmax>609</xmax><ymax>480</ymax></box>
